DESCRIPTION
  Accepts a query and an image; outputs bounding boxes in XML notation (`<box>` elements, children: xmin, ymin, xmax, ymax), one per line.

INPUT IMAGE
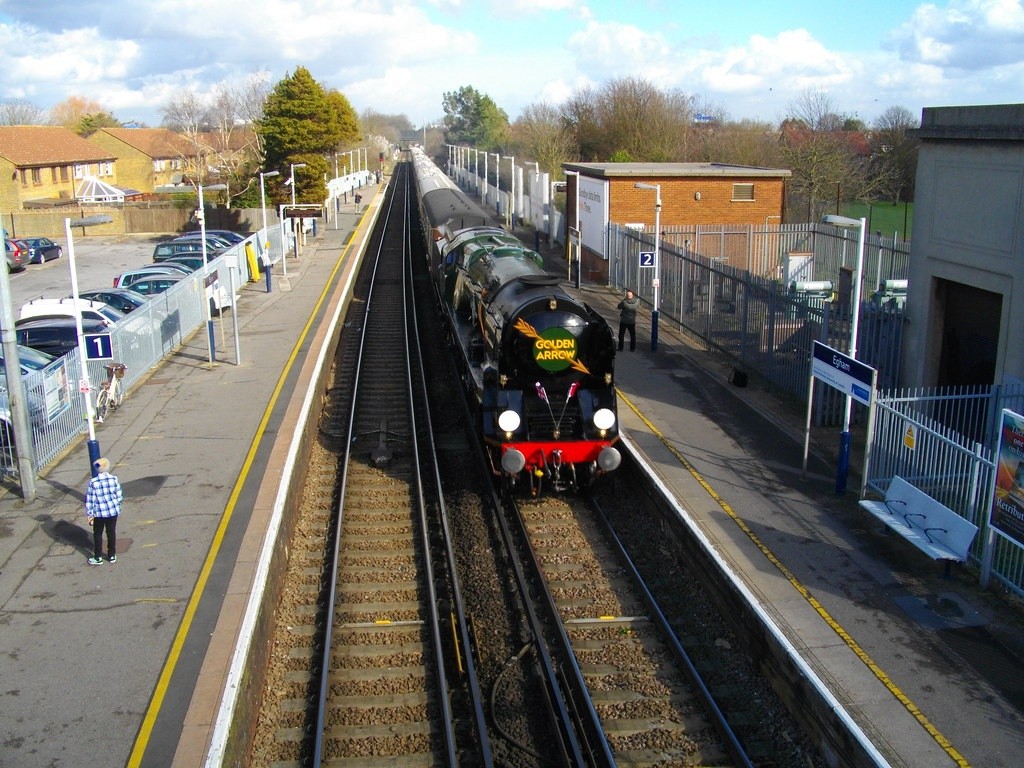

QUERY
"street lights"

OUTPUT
<box><xmin>283</xmin><ymin>163</ymin><xmax>307</xmax><ymax>259</ymax></box>
<box><xmin>524</xmin><ymin>161</ymin><xmax>540</xmax><ymax>253</ymax></box>
<box><xmin>562</xmin><ymin>170</ymin><xmax>580</xmax><ymax>289</ymax></box>
<box><xmin>66</xmin><ymin>215</ymin><xmax>115</xmax><ymax>469</ymax></box>
<box><xmin>469</xmin><ymin>148</ymin><xmax>478</xmax><ymax>198</ymax></box>
<box><xmin>445</xmin><ymin>144</ymin><xmax>470</xmax><ymax>191</ymax></box>
<box><xmin>259</xmin><ymin>170</ymin><xmax>280</xmax><ymax>292</ymax></box>
<box><xmin>189</xmin><ymin>184</ymin><xmax>227</xmax><ymax>363</ymax></box>
<box><xmin>479</xmin><ymin>151</ymin><xmax>488</xmax><ymax>207</ymax></box>
<box><xmin>489</xmin><ymin>153</ymin><xmax>500</xmax><ymax>217</ymax></box>
<box><xmin>501</xmin><ymin>155</ymin><xmax>515</xmax><ymax>230</ymax></box>
<box><xmin>322</xmin><ymin>145</ymin><xmax>371</xmax><ymax>221</ymax></box>
<box><xmin>822</xmin><ymin>213</ymin><xmax>866</xmax><ymax>490</ymax></box>
<box><xmin>633</xmin><ymin>183</ymin><xmax>662</xmax><ymax>350</ymax></box>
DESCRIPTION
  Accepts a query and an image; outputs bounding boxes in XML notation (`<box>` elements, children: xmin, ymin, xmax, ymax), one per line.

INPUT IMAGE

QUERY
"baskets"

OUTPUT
<box><xmin>107</xmin><ymin>363</ymin><xmax>125</xmax><ymax>379</ymax></box>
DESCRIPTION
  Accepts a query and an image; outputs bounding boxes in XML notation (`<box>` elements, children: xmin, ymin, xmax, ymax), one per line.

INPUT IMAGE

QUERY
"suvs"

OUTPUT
<box><xmin>9</xmin><ymin>315</ymin><xmax>139</xmax><ymax>375</ymax></box>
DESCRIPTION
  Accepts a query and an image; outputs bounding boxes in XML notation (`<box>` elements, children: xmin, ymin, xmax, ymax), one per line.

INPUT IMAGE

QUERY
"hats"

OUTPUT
<box><xmin>93</xmin><ymin>458</ymin><xmax>110</xmax><ymax>473</ymax></box>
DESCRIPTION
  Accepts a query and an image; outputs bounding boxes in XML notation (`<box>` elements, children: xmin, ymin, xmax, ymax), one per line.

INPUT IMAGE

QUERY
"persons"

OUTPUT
<box><xmin>376</xmin><ymin>170</ymin><xmax>380</xmax><ymax>184</ymax></box>
<box><xmin>617</xmin><ymin>291</ymin><xmax>638</xmax><ymax>352</ymax></box>
<box><xmin>355</xmin><ymin>192</ymin><xmax>363</xmax><ymax>214</ymax></box>
<box><xmin>86</xmin><ymin>458</ymin><xmax>123</xmax><ymax>565</ymax></box>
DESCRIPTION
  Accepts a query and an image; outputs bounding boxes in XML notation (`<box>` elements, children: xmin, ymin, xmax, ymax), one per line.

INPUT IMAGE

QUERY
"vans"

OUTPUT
<box><xmin>20</xmin><ymin>297</ymin><xmax>162</xmax><ymax>362</ymax></box>
<box><xmin>152</xmin><ymin>229</ymin><xmax>257</xmax><ymax>272</ymax></box>
<box><xmin>0</xmin><ymin>339</ymin><xmax>72</xmax><ymax>450</ymax></box>
<box><xmin>4</xmin><ymin>234</ymin><xmax>32</xmax><ymax>273</ymax></box>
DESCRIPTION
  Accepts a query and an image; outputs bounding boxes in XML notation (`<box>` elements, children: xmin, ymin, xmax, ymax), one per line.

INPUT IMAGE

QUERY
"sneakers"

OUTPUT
<box><xmin>87</xmin><ymin>557</ymin><xmax>104</xmax><ymax>566</ymax></box>
<box><xmin>107</xmin><ymin>555</ymin><xmax>118</xmax><ymax>564</ymax></box>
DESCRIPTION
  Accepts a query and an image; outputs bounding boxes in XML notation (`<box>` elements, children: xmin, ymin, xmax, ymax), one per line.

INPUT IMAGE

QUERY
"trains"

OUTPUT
<box><xmin>408</xmin><ymin>142</ymin><xmax>621</xmax><ymax>500</ymax></box>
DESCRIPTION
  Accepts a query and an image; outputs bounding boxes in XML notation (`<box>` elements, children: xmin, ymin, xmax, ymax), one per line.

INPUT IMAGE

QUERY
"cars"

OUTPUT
<box><xmin>68</xmin><ymin>251</ymin><xmax>228</xmax><ymax>339</ymax></box>
<box><xmin>23</xmin><ymin>236</ymin><xmax>63</xmax><ymax>264</ymax></box>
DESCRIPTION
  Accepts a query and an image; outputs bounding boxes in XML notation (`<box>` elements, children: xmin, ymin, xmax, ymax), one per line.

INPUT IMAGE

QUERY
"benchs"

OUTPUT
<box><xmin>212</xmin><ymin>286</ymin><xmax>241</xmax><ymax>309</ymax></box>
<box><xmin>259</xmin><ymin>254</ymin><xmax>280</xmax><ymax>268</ymax></box>
<box><xmin>858</xmin><ymin>475</ymin><xmax>979</xmax><ymax>578</ymax></box>
